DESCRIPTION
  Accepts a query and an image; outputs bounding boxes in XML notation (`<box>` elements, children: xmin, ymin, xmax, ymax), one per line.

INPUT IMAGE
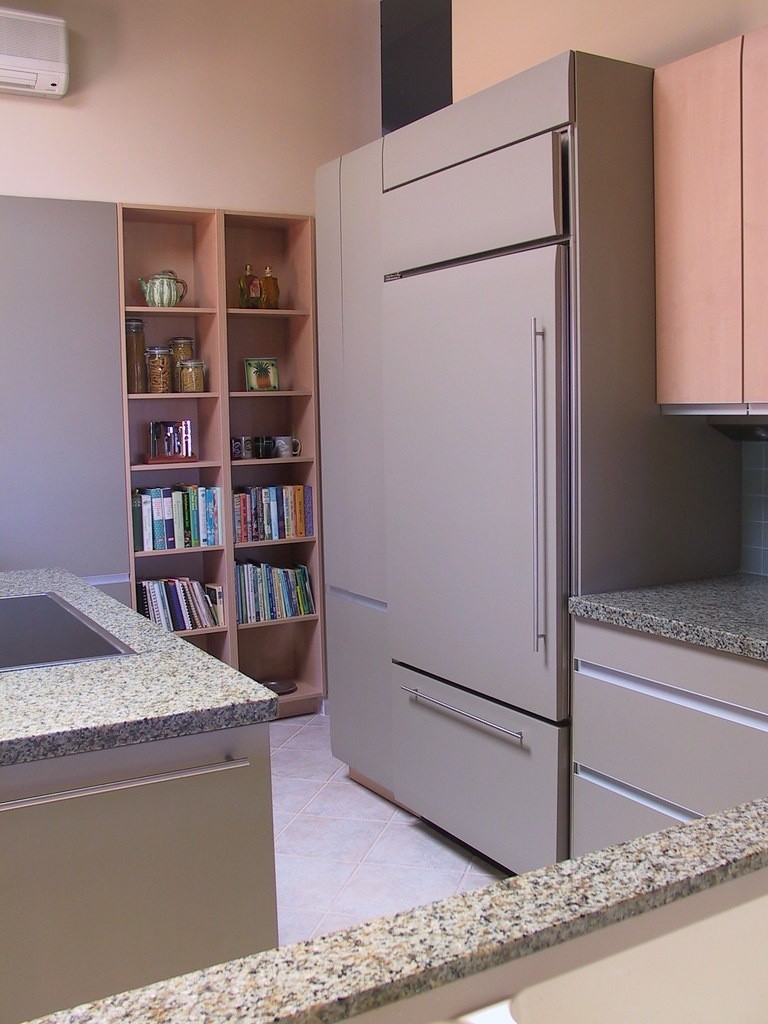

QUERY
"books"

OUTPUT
<box><xmin>131</xmin><ymin>482</ymin><xmax>223</xmax><ymax>552</ymax></box>
<box><xmin>136</xmin><ymin>577</ymin><xmax>225</xmax><ymax>632</ymax></box>
<box><xmin>233</xmin><ymin>484</ymin><xmax>314</xmax><ymax>543</ymax></box>
<box><xmin>234</xmin><ymin>561</ymin><xmax>315</xmax><ymax>624</ymax></box>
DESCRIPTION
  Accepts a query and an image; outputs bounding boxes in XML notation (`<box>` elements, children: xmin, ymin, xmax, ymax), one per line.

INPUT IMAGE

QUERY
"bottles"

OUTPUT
<box><xmin>260</xmin><ymin>266</ymin><xmax>280</xmax><ymax>309</ymax></box>
<box><xmin>238</xmin><ymin>265</ymin><xmax>260</xmax><ymax>309</ymax></box>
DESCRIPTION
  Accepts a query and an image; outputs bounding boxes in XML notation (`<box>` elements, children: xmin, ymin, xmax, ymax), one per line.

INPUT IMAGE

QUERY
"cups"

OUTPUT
<box><xmin>240</xmin><ymin>436</ymin><xmax>252</xmax><ymax>458</ymax></box>
<box><xmin>230</xmin><ymin>439</ymin><xmax>241</xmax><ymax>460</ymax></box>
<box><xmin>255</xmin><ymin>436</ymin><xmax>277</xmax><ymax>459</ymax></box>
<box><xmin>275</xmin><ymin>436</ymin><xmax>300</xmax><ymax>457</ymax></box>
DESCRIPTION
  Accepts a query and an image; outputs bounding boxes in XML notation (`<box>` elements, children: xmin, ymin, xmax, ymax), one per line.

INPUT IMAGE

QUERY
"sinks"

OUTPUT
<box><xmin>0</xmin><ymin>589</ymin><xmax>139</xmax><ymax>672</ymax></box>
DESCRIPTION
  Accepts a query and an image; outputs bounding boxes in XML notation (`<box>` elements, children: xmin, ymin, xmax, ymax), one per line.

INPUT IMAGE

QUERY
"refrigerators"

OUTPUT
<box><xmin>315</xmin><ymin>50</ymin><xmax>742</xmax><ymax>875</ymax></box>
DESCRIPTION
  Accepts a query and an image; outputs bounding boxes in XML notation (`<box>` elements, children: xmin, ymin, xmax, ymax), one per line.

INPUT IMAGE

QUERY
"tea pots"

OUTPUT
<box><xmin>137</xmin><ymin>270</ymin><xmax>187</xmax><ymax>307</ymax></box>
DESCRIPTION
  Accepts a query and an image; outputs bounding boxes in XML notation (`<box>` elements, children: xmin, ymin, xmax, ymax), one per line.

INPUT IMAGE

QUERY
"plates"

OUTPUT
<box><xmin>262</xmin><ymin>679</ymin><xmax>297</xmax><ymax>694</ymax></box>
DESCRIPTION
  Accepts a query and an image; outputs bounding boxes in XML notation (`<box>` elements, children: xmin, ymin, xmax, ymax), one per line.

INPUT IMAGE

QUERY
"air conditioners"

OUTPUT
<box><xmin>0</xmin><ymin>10</ymin><xmax>68</xmax><ymax>101</ymax></box>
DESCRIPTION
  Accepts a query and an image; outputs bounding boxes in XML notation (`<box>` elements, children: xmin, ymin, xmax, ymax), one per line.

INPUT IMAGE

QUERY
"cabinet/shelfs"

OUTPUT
<box><xmin>115</xmin><ymin>202</ymin><xmax>325</xmax><ymax>724</ymax></box>
<box><xmin>572</xmin><ymin>630</ymin><xmax>767</xmax><ymax>872</ymax></box>
